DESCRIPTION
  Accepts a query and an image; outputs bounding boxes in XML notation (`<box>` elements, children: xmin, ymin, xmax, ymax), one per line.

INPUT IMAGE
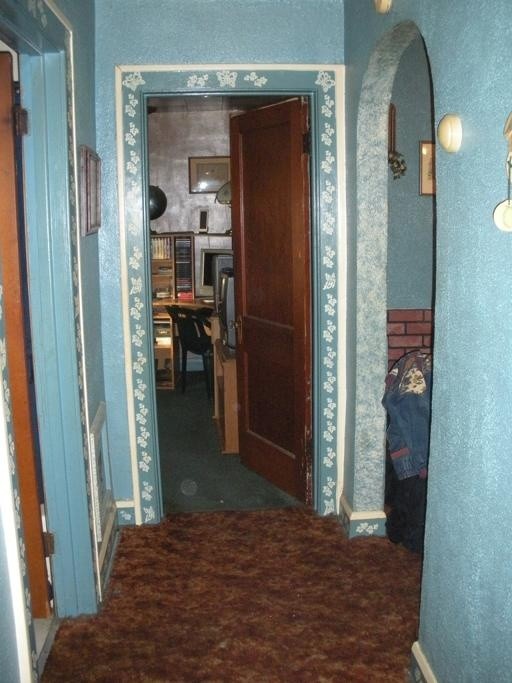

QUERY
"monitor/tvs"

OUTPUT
<box><xmin>201</xmin><ymin>249</ymin><xmax>234</xmax><ymax>303</ymax></box>
<box><xmin>217</xmin><ymin>270</ymin><xmax>235</xmax><ymax>349</ymax></box>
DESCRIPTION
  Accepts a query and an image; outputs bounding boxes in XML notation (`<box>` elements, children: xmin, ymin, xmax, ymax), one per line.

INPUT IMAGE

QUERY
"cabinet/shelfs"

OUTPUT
<box><xmin>149</xmin><ymin>231</ymin><xmax>196</xmax><ymax>299</ymax></box>
<box><xmin>213</xmin><ymin>338</ymin><xmax>238</xmax><ymax>455</ymax></box>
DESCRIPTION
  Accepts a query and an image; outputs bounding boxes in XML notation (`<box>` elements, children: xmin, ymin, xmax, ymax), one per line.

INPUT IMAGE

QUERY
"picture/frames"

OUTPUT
<box><xmin>417</xmin><ymin>139</ymin><xmax>435</xmax><ymax>195</ymax></box>
<box><xmin>188</xmin><ymin>155</ymin><xmax>232</xmax><ymax>194</ymax></box>
<box><xmin>76</xmin><ymin>143</ymin><xmax>101</xmax><ymax>236</ymax></box>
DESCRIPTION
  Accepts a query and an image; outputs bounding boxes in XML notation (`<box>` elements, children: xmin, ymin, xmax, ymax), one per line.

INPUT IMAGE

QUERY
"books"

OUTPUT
<box><xmin>151</xmin><ymin>236</ymin><xmax>170</xmax><ymax>260</ymax></box>
<box><xmin>154</xmin><ymin>326</ymin><xmax>171</xmax><ymax>345</ymax></box>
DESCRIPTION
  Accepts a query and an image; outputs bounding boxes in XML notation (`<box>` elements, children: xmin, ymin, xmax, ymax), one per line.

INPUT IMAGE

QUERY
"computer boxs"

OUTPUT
<box><xmin>211</xmin><ymin>255</ymin><xmax>235</xmax><ymax>312</ymax></box>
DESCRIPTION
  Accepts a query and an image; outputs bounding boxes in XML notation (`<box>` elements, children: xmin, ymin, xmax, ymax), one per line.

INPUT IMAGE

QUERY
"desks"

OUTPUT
<box><xmin>151</xmin><ymin>297</ymin><xmax>218</xmax><ymax>422</ymax></box>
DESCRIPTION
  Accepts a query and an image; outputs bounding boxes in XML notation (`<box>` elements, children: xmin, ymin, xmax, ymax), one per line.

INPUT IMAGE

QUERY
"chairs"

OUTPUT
<box><xmin>164</xmin><ymin>304</ymin><xmax>214</xmax><ymax>401</ymax></box>
